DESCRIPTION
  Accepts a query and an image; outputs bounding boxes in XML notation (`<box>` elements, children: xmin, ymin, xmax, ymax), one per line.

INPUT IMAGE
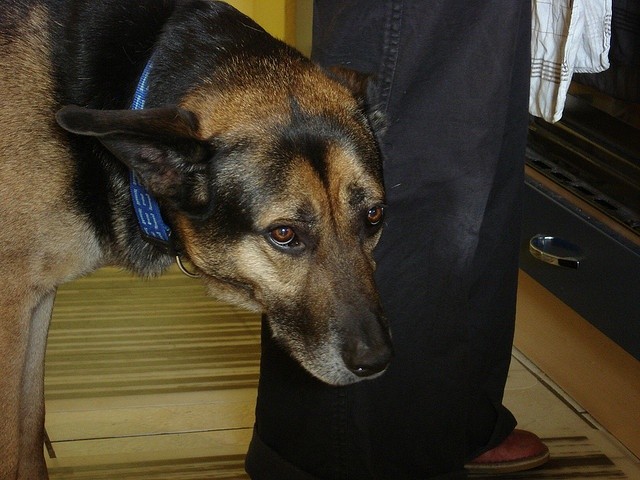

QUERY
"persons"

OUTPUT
<box><xmin>243</xmin><ymin>0</ymin><xmax>553</xmax><ymax>480</ymax></box>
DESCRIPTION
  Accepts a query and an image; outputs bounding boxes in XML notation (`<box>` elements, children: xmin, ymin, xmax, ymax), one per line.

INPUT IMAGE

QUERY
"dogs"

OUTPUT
<box><xmin>0</xmin><ymin>1</ymin><xmax>397</xmax><ymax>480</ymax></box>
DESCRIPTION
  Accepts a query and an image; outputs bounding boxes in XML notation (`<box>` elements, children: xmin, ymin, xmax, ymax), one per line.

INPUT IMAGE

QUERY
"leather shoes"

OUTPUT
<box><xmin>463</xmin><ymin>429</ymin><xmax>550</xmax><ymax>475</ymax></box>
